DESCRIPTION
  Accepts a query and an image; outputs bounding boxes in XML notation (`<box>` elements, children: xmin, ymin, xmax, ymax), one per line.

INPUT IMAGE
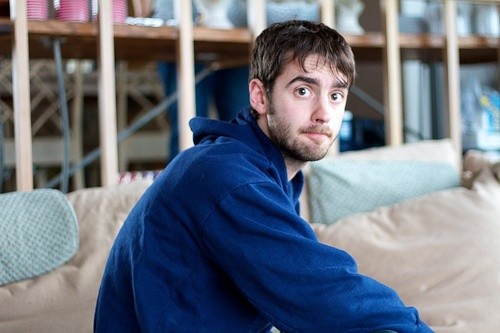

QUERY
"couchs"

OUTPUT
<box><xmin>1</xmin><ymin>138</ymin><xmax>500</xmax><ymax>333</ymax></box>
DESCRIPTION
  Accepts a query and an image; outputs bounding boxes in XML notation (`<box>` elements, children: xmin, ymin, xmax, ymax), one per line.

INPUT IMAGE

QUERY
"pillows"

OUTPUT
<box><xmin>1</xmin><ymin>187</ymin><xmax>81</xmax><ymax>286</ymax></box>
<box><xmin>305</xmin><ymin>138</ymin><xmax>462</xmax><ymax>228</ymax></box>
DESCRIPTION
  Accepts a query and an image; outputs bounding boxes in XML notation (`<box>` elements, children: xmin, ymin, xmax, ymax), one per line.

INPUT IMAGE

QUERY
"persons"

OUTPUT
<box><xmin>92</xmin><ymin>19</ymin><xmax>440</xmax><ymax>332</ymax></box>
